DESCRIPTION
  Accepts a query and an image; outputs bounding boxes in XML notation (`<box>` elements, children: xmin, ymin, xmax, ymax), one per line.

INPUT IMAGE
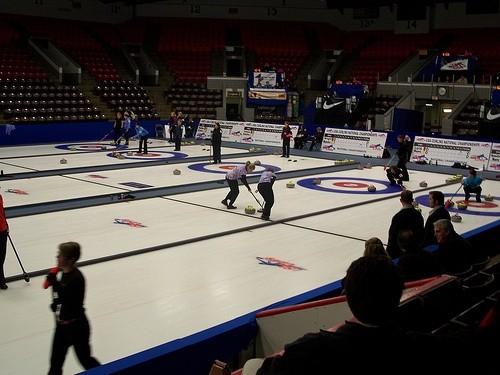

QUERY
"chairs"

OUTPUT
<box><xmin>0</xmin><ymin>44</ymin><xmax>487</xmax><ymax>134</ymax></box>
<box><xmin>429</xmin><ymin>248</ymin><xmax>500</xmax><ymax>334</ymax></box>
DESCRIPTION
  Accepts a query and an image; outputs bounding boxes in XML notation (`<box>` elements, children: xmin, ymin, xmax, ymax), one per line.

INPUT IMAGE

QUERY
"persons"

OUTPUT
<box><xmin>253</xmin><ymin>189</ymin><xmax>479</xmax><ymax>375</ymax></box>
<box><xmin>461</xmin><ymin>168</ymin><xmax>483</xmax><ymax>204</ymax></box>
<box><xmin>210</xmin><ymin>122</ymin><xmax>222</xmax><ymax>164</ymax></box>
<box><xmin>282</xmin><ymin>120</ymin><xmax>293</xmax><ymax>158</ymax></box>
<box><xmin>256</xmin><ymin>167</ymin><xmax>277</xmax><ymax>220</ymax></box>
<box><xmin>386</xmin><ymin>134</ymin><xmax>414</xmax><ymax>187</ymax></box>
<box><xmin>168</xmin><ymin>111</ymin><xmax>194</xmax><ymax>151</ymax></box>
<box><xmin>308</xmin><ymin>127</ymin><xmax>324</xmax><ymax>151</ymax></box>
<box><xmin>222</xmin><ymin>160</ymin><xmax>255</xmax><ymax>209</ymax></box>
<box><xmin>47</xmin><ymin>241</ymin><xmax>101</xmax><ymax>375</ymax></box>
<box><xmin>130</xmin><ymin>123</ymin><xmax>150</xmax><ymax>154</ymax></box>
<box><xmin>294</xmin><ymin>126</ymin><xmax>307</xmax><ymax>149</ymax></box>
<box><xmin>0</xmin><ymin>194</ymin><xmax>9</xmax><ymax>290</ymax></box>
<box><xmin>114</xmin><ymin>112</ymin><xmax>131</xmax><ymax>145</ymax></box>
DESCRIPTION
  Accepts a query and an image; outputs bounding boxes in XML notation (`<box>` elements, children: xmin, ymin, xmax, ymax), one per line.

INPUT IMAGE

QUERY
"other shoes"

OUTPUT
<box><xmin>137</xmin><ymin>151</ymin><xmax>141</xmax><ymax>153</ymax></box>
<box><xmin>0</xmin><ymin>282</ymin><xmax>8</xmax><ymax>289</ymax></box>
<box><xmin>144</xmin><ymin>151</ymin><xmax>147</xmax><ymax>154</ymax></box>
<box><xmin>221</xmin><ymin>200</ymin><xmax>228</xmax><ymax>206</ymax></box>
<box><xmin>261</xmin><ymin>216</ymin><xmax>270</xmax><ymax>220</ymax></box>
<box><xmin>228</xmin><ymin>204</ymin><xmax>237</xmax><ymax>209</ymax></box>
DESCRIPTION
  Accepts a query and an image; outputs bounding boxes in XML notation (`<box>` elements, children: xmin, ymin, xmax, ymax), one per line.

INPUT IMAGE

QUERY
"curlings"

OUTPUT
<box><xmin>101</xmin><ymin>145</ymin><xmax>106</xmax><ymax>150</ymax></box>
<box><xmin>173</xmin><ymin>169</ymin><xmax>181</xmax><ymax>175</ymax></box>
<box><xmin>334</xmin><ymin>159</ymin><xmax>355</xmax><ymax>165</ymax></box>
<box><xmin>286</xmin><ymin>181</ymin><xmax>295</xmax><ymax>188</ymax></box>
<box><xmin>110</xmin><ymin>142</ymin><xmax>114</xmax><ymax>146</ymax></box>
<box><xmin>60</xmin><ymin>158</ymin><xmax>67</xmax><ymax>164</ymax></box>
<box><xmin>312</xmin><ymin>178</ymin><xmax>321</xmax><ymax>184</ymax></box>
<box><xmin>445</xmin><ymin>174</ymin><xmax>463</xmax><ymax>184</ymax></box>
<box><xmin>114</xmin><ymin>151</ymin><xmax>133</xmax><ymax>159</ymax></box>
<box><xmin>452</xmin><ymin>214</ymin><xmax>461</xmax><ymax>222</ymax></box>
<box><xmin>249</xmin><ymin>146</ymin><xmax>261</xmax><ymax>152</ymax></box>
<box><xmin>368</xmin><ymin>185</ymin><xmax>376</xmax><ymax>192</ymax></box>
<box><xmin>485</xmin><ymin>194</ymin><xmax>493</xmax><ymax>201</ymax></box>
<box><xmin>71</xmin><ymin>146</ymin><xmax>76</xmax><ymax>151</ymax></box>
<box><xmin>358</xmin><ymin>163</ymin><xmax>372</xmax><ymax>170</ymax></box>
<box><xmin>458</xmin><ymin>202</ymin><xmax>467</xmax><ymax>209</ymax></box>
<box><xmin>412</xmin><ymin>201</ymin><xmax>422</xmax><ymax>212</ymax></box>
<box><xmin>420</xmin><ymin>181</ymin><xmax>427</xmax><ymax>187</ymax></box>
<box><xmin>245</xmin><ymin>205</ymin><xmax>255</xmax><ymax>214</ymax></box>
<box><xmin>181</xmin><ymin>141</ymin><xmax>195</xmax><ymax>145</ymax></box>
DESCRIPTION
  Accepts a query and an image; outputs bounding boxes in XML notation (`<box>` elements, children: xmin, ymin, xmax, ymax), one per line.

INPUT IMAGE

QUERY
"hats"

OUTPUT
<box><xmin>135</xmin><ymin>123</ymin><xmax>139</xmax><ymax>125</ymax></box>
